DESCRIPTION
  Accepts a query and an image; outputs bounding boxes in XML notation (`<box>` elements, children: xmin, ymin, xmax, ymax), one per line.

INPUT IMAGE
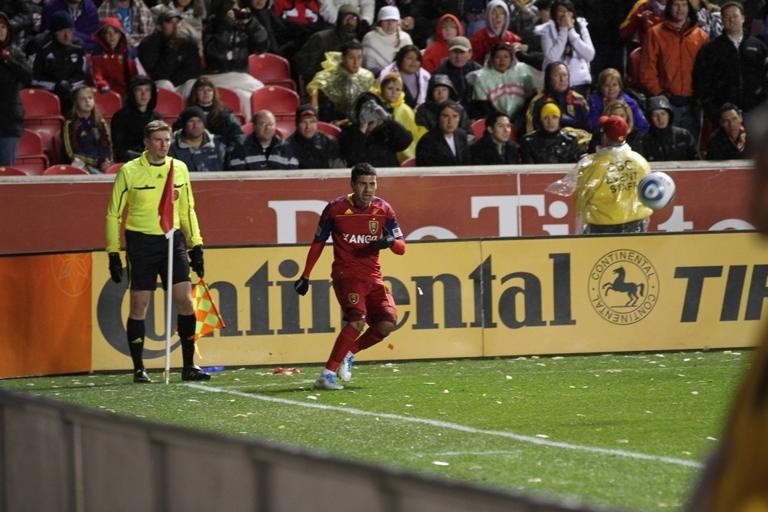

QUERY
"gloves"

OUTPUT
<box><xmin>108</xmin><ymin>252</ymin><xmax>125</xmax><ymax>284</ymax></box>
<box><xmin>369</xmin><ymin>234</ymin><xmax>395</xmax><ymax>251</ymax></box>
<box><xmin>294</xmin><ymin>276</ymin><xmax>310</xmax><ymax>296</ymax></box>
<box><xmin>187</xmin><ymin>245</ymin><xmax>205</xmax><ymax>279</ymax></box>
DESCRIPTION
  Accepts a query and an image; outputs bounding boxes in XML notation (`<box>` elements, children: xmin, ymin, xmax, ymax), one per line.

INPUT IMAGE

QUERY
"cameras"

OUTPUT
<box><xmin>365</xmin><ymin>101</ymin><xmax>373</xmax><ymax>114</ymax></box>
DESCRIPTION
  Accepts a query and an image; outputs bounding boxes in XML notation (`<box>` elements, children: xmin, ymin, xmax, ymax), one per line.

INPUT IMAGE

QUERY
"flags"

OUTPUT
<box><xmin>191</xmin><ymin>280</ymin><xmax>224</xmax><ymax>359</ymax></box>
<box><xmin>157</xmin><ymin>160</ymin><xmax>174</xmax><ymax>239</ymax></box>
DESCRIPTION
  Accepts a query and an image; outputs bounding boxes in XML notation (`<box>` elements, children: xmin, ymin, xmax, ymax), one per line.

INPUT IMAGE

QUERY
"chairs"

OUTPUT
<box><xmin>0</xmin><ymin>52</ymin><xmax>513</xmax><ymax>176</ymax></box>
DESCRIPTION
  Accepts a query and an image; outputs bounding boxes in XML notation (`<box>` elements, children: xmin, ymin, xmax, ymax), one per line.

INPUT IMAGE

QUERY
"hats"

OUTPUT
<box><xmin>156</xmin><ymin>10</ymin><xmax>183</xmax><ymax>24</ymax></box>
<box><xmin>378</xmin><ymin>5</ymin><xmax>400</xmax><ymax>23</ymax></box>
<box><xmin>540</xmin><ymin>102</ymin><xmax>561</xmax><ymax>120</ymax></box>
<box><xmin>598</xmin><ymin>114</ymin><xmax>629</xmax><ymax>142</ymax></box>
<box><xmin>50</xmin><ymin>10</ymin><xmax>75</xmax><ymax>30</ymax></box>
<box><xmin>180</xmin><ymin>106</ymin><xmax>207</xmax><ymax>126</ymax></box>
<box><xmin>295</xmin><ymin>104</ymin><xmax>317</xmax><ymax>119</ymax></box>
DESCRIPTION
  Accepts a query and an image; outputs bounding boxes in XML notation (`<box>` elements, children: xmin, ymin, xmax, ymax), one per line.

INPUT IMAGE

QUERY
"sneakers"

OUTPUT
<box><xmin>133</xmin><ymin>369</ymin><xmax>152</xmax><ymax>384</ymax></box>
<box><xmin>182</xmin><ymin>364</ymin><xmax>211</xmax><ymax>381</ymax></box>
<box><xmin>313</xmin><ymin>371</ymin><xmax>344</xmax><ymax>391</ymax></box>
<box><xmin>337</xmin><ymin>351</ymin><xmax>355</xmax><ymax>382</ymax></box>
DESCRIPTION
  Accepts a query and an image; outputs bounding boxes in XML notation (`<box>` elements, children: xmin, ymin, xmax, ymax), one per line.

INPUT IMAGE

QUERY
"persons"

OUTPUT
<box><xmin>572</xmin><ymin>114</ymin><xmax>655</xmax><ymax>236</ymax></box>
<box><xmin>104</xmin><ymin>119</ymin><xmax>213</xmax><ymax>383</ymax></box>
<box><xmin>293</xmin><ymin>161</ymin><xmax>407</xmax><ymax>390</ymax></box>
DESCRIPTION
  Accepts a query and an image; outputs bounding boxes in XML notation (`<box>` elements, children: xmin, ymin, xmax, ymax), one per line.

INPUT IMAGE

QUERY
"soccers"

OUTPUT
<box><xmin>637</xmin><ymin>171</ymin><xmax>677</xmax><ymax>211</ymax></box>
